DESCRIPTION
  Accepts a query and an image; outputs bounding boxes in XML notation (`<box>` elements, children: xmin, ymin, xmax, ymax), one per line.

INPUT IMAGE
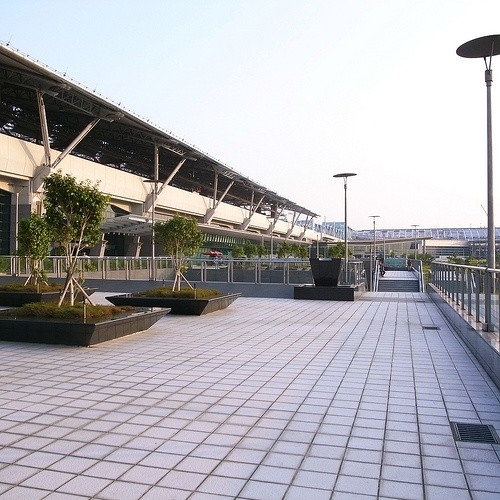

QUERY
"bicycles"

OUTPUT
<box><xmin>379</xmin><ymin>263</ymin><xmax>386</xmax><ymax>278</ymax></box>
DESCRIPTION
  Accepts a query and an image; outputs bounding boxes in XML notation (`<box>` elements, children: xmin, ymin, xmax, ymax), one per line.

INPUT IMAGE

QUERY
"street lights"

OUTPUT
<box><xmin>333</xmin><ymin>173</ymin><xmax>358</xmax><ymax>284</ymax></box>
<box><xmin>143</xmin><ymin>180</ymin><xmax>161</xmax><ymax>281</ymax></box>
<box><xmin>369</xmin><ymin>215</ymin><xmax>380</xmax><ymax>273</ymax></box>
<box><xmin>455</xmin><ymin>33</ymin><xmax>500</xmax><ymax>277</ymax></box>
<box><xmin>411</xmin><ymin>224</ymin><xmax>419</xmax><ymax>260</ymax></box>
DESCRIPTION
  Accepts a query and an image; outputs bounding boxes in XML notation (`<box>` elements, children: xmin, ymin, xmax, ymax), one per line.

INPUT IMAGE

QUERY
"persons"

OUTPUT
<box><xmin>406</xmin><ymin>257</ymin><xmax>412</xmax><ymax>272</ymax></box>
<box><xmin>379</xmin><ymin>256</ymin><xmax>386</xmax><ymax>277</ymax></box>
